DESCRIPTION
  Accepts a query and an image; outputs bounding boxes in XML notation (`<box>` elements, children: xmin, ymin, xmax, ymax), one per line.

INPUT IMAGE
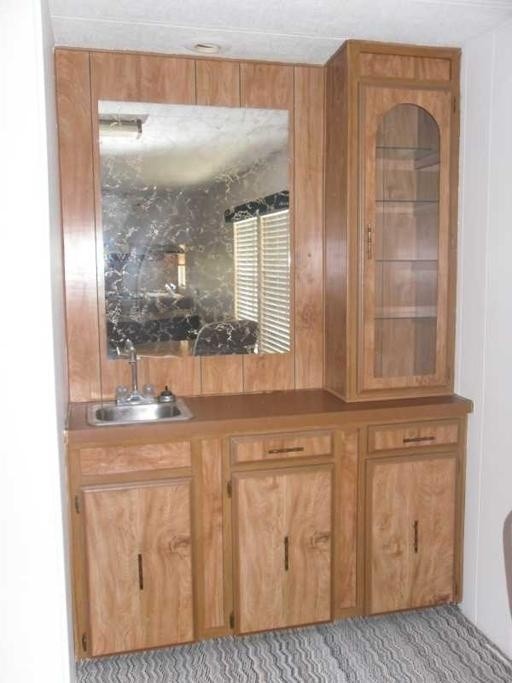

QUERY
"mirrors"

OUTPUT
<box><xmin>55</xmin><ymin>47</ymin><xmax>324</xmax><ymax>402</ymax></box>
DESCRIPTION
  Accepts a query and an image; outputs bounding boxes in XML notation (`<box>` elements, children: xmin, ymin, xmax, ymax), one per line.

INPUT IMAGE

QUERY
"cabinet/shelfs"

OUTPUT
<box><xmin>322</xmin><ymin>37</ymin><xmax>461</xmax><ymax>404</ymax></box>
<box><xmin>65</xmin><ymin>415</ymin><xmax>465</xmax><ymax>662</ymax></box>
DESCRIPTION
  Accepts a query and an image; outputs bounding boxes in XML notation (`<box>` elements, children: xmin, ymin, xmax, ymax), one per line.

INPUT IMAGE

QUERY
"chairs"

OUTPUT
<box><xmin>192</xmin><ymin>319</ymin><xmax>258</xmax><ymax>356</ymax></box>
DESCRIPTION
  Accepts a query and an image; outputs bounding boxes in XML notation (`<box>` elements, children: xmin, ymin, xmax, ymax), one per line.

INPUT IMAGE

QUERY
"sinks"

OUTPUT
<box><xmin>85</xmin><ymin>395</ymin><xmax>195</xmax><ymax>426</ymax></box>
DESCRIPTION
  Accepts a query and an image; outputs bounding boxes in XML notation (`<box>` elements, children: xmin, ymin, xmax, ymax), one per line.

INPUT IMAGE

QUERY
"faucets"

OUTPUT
<box><xmin>122</xmin><ymin>338</ymin><xmax>134</xmax><ymax>354</ymax></box>
<box><xmin>128</xmin><ymin>346</ymin><xmax>138</xmax><ymax>393</ymax></box>
